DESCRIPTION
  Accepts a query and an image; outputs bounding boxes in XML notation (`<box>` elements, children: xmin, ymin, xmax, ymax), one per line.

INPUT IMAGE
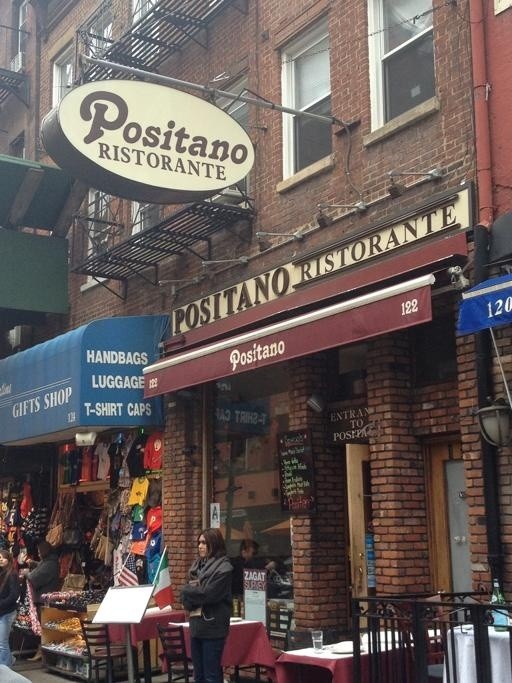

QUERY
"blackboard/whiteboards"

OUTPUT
<box><xmin>276</xmin><ymin>428</ymin><xmax>317</xmax><ymax>516</ymax></box>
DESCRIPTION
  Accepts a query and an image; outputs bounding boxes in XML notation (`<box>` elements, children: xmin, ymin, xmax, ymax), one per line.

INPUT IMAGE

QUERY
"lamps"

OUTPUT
<box><xmin>73</xmin><ymin>430</ymin><xmax>98</xmax><ymax>445</ymax></box>
<box><xmin>472</xmin><ymin>394</ymin><xmax>512</xmax><ymax>447</ymax></box>
<box><xmin>157</xmin><ymin>168</ymin><xmax>443</xmax><ymax>298</ymax></box>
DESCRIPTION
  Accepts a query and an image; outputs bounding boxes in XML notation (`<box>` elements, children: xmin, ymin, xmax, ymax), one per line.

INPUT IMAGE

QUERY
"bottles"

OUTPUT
<box><xmin>488</xmin><ymin>577</ymin><xmax>511</xmax><ymax>632</ymax></box>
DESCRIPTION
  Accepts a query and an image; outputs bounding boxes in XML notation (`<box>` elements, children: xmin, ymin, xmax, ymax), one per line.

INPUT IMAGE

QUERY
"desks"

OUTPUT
<box><xmin>163</xmin><ymin>617</ymin><xmax>276</xmax><ymax>680</ymax></box>
<box><xmin>86</xmin><ymin>609</ymin><xmax>186</xmax><ymax>681</ymax></box>
<box><xmin>275</xmin><ymin>590</ymin><xmax>509</xmax><ymax>683</ymax></box>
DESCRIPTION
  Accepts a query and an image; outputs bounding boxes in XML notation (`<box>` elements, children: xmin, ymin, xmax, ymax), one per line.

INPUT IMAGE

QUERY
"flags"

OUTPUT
<box><xmin>152</xmin><ymin>551</ymin><xmax>176</xmax><ymax>610</ymax></box>
<box><xmin>118</xmin><ymin>549</ymin><xmax>140</xmax><ymax>586</ymax></box>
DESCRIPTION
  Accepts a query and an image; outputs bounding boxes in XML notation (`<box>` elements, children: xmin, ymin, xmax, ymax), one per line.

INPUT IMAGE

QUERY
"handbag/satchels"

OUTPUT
<box><xmin>90</xmin><ymin>526</ymin><xmax>115</xmax><ymax>567</ymax></box>
<box><xmin>62</xmin><ymin>574</ymin><xmax>86</xmax><ymax>591</ymax></box>
<box><xmin>46</xmin><ymin>510</ymin><xmax>81</xmax><ymax>548</ymax></box>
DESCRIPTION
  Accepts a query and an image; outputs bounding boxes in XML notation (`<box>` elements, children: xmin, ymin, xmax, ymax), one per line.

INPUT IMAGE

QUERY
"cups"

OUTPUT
<box><xmin>311</xmin><ymin>629</ymin><xmax>325</xmax><ymax>651</ymax></box>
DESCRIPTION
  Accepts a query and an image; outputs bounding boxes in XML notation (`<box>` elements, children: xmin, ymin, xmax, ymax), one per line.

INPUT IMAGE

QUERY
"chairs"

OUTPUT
<box><xmin>87</xmin><ymin>604</ymin><xmax>139</xmax><ymax>680</ymax></box>
<box><xmin>234</xmin><ymin>606</ymin><xmax>294</xmax><ymax>682</ymax></box>
<box><xmin>79</xmin><ymin>617</ymin><xmax>128</xmax><ymax>682</ymax></box>
<box><xmin>154</xmin><ymin>620</ymin><xmax>193</xmax><ymax>681</ymax></box>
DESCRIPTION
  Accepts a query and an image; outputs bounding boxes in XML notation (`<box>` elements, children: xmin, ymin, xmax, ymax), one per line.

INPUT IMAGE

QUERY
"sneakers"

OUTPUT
<box><xmin>11</xmin><ymin>656</ymin><xmax>16</xmax><ymax>665</ymax></box>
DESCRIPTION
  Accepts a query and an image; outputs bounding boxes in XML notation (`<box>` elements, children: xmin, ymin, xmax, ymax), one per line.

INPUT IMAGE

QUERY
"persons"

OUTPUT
<box><xmin>180</xmin><ymin>527</ymin><xmax>236</xmax><ymax>682</ymax></box>
<box><xmin>229</xmin><ymin>537</ymin><xmax>262</xmax><ymax>599</ymax></box>
<box><xmin>23</xmin><ymin>539</ymin><xmax>60</xmax><ymax>661</ymax></box>
<box><xmin>0</xmin><ymin>548</ymin><xmax>20</xmax><ymax>667</ymax></box>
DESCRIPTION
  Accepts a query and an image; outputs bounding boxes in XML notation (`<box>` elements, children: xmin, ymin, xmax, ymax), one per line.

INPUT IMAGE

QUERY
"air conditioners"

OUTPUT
<box><xmin>10</xmin><ymin>51</ymin><xmax>25</xmax><ymax>73</ymax></box>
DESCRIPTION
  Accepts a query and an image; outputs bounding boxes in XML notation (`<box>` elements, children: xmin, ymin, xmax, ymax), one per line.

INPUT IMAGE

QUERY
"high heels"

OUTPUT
<box><xmin>26</xmin><ymin>653</ymin><xmax>43</xmax><ymax>663</ymax></box>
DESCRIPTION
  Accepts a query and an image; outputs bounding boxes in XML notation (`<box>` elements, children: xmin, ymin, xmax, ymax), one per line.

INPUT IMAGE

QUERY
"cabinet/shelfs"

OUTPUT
<box><xmin>39</xmin><ymin>603</ymin><xmax>130</xmax><ymax>680</ymax></box>
